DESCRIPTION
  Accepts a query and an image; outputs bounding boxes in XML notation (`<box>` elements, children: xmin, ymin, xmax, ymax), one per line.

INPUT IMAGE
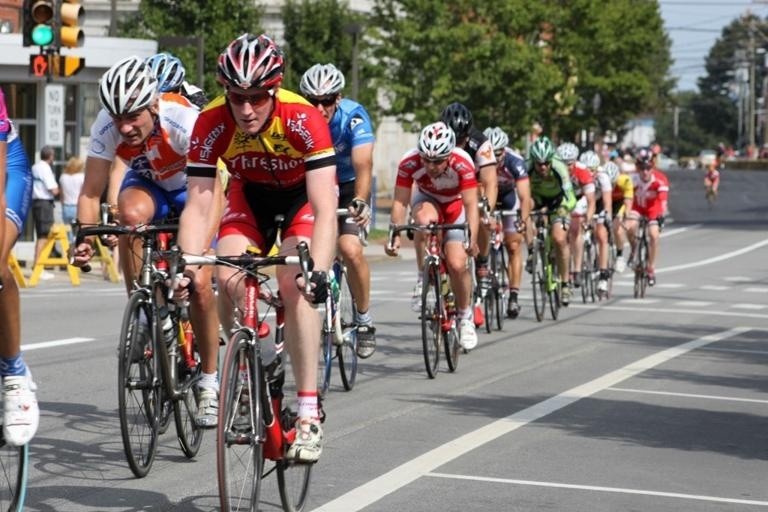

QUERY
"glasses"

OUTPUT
<box><xmin>225</xmin><ymin>90</ymin><xmax>269</xmax><ymax>107</ymax></box>
<box><xmin>421</xmin><ymin>157</ymin><xmax>445</xmax><ymax>166</ymax></box>
<box><xmin>310</xmin><ymin>94</ymin><xmax>335</xmax><ymax>107</ymax></box>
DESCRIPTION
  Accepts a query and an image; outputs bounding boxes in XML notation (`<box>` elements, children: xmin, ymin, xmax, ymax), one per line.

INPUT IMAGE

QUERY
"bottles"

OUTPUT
<box><xmin>443</xmin><ymin>292</ymin><xmax>456</xmax><ymax>329</ymax></box>
<box><xmin>255</xmin><ymin>322</ymin><xmax>276</xmax><ymax>366</ymax></box>
<box><xmin>167</xmin><ymin>343</ymin><xmax>179</xmax><ymax>385</ymax></box>
<box><xmin>159</xmin><ymin>305</ymin><xmax>174</xmax><ymax>346</ymax></box>
<box><xmin>329</xmin><ymin>272</ymin><xmax>340</xmax><ymax>304</ymax></box>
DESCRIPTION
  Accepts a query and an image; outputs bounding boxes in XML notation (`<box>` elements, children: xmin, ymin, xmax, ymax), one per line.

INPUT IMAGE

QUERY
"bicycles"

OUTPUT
<box><xmin>383</xmin><ymin>221</ymin><xmax>472</xmax><ymax>381</ymax></box>
<box><xmin>276</xmin><ymin>201</ymin><xmax>369</xmax><ymax>402</ymax></box>
<box><xmin>153</xmin><ymin>216</ymin><xmax>323</xmax><ymax>512</ymax></box>
<box><xmin>706</xmin><ymin>184</ymin><xmax>715</xmax><ymax>214</ymax></box>
<box><xmin>68</xmin><ymin>219</ymin><xmax>213</xmax><ymax>479</ymax></box>
<box><xmin>1</xmin><ymin>427</ymin><xmax>33</xmax><ymax>512</ymax></box>
<box><xmin>478</xmin><ymin>207</ymin><xmax>662</xmax><ymax>336</ymax></box>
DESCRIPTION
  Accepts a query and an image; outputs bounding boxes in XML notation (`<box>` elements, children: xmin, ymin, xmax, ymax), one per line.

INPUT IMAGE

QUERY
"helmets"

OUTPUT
<box><xmin>533</xmin><ymin>137</ymin><xmax>600</xmax><ymax>169</ymax></box>
<box><xmin>217</xmin><ymin>34</ymin><xmax>285</xmax><ymax>91</ymax></box>
<box><xmin>483</xmin><ymin>128</ymin><xmax>509</xmax><ymax>151</ymax></box>
<box><xmin>636</xmin><ymin>149</ymin><xmax>656</xmax><ymax>167</ymax></box>
<box><xmin>300</xmin><ymin>63</ymin><xmax>345</xmax><ymax>98</ymax></box>
<box><xmin>603</xmin><ymin>162</ymin><xmax>619</xmax><ymax>180</ymax></box>
<box><xmin>417</xmin><ymin>103</ymin><xmax>473</xmax><ymax>160</ymax></box>
<box><xmin>99</xmin><ymin>52</ymin><xmax>185</xmax><ymax>119</ymax></box>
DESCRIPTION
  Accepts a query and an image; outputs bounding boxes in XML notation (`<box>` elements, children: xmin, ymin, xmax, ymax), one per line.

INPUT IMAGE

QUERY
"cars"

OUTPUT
<box><xmin>657</xmin><ymin>152</ymin><xmax>680</xmax><ymax>170</ymax></box>
<box><xmin>696</xmin><ymin>148</ymin><xmax>717</xmax><ymax>169</ymax></box>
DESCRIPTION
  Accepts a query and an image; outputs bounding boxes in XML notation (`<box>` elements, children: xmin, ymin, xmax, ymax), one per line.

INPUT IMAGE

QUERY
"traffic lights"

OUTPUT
<box><xmin>52</xmin><ymin>55</ymin><xmax>85</xmax><ymax>79</ymax></box>
<box><xmin>28</xmin><ymin>54</ymin><xmax>54</xmax><ymax>78</ymax></box>
<box><xmin>22</xmin><ymin>1</ymin><xmax>61</xmax><ymax>49</ymax></box>
<box><xmin>54</xmin><ymin>0</ymin><xmax>86</xmax><ymax>50</ymax></box>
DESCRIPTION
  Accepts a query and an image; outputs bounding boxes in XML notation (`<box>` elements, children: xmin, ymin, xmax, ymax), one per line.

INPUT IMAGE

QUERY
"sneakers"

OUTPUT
<box><xmin>116</xmin><ymin>322</ymin><xmax>151</xmax><ymax>362</ymax></box>
<box><xmin>356</xmin><ymin>323</ymin><xmax>376</xmax><ymax>359</ymax></box>
<box><xmin>458</xmin><ymin>318</ymin><xmax>478</xmax><ymax>351</ymax></box>
<box><xmin>195</xmin><ymin>381</ymin><xmax>222</xmax><ymax>429</ymax></box>
<box><xmin>411</xmin><ymin>283</ymin><xmax>425</xmax><ymax>312</ymax></box>
<box><xmin>288</xmin><ymin>420</ymin><xmax>324</xmax><ymax>462</ymax></box>
<box><xmin>560</xmin><ymin>282</ymin><xmax>571</xmax><ymax>306</ymax></box>
<box><xmin>596</xmin><ymin>278</ymin><xmax>608</xmax><ymax>292</ymax></box>
<box><xmin>647</xmin><ymin>268</ymin><xmax>656</xmax><ymax>286</ymax></box>
<box><xmin>0</xmin><ymin>370</ymin><xmax>40</xmax><ymax>446</ymax></box>
<box><xmin>506</xmin><ymin>300</ymin><xmax>518</xmax><ymax>318</ymax></box>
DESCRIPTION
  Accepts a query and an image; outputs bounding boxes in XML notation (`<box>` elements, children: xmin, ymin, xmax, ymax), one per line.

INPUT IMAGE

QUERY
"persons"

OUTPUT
<box><xmin>298</xmin><ymin>61</ymin><xmax>376</xmax><ymax>359</ymax></box>
<box><xmin>102</xmin><ymin>54</ymin><xmax>212</xmax><ymax>251</ymax></box>
<box><xmin>166</xmin><ymin>33</ymin><xmax>340</xmax><ymax>464</ymax></box>
<box><xmin>0</xmin><ymin>90</ymin><xmax>40</xmax><ymax>448</ymax></box>
<box><xmin>30</xmin><ymin>144</ymin><xmax>60</xmax><ymax>271</ymax></box>
<box><xmin>57</xmin><ymin>156</ymin><xmax>85</xmax><ymax>243</ymax></box>
<box><xmin>63</xmin><ymin>57</ymin><xmax>220</xmax><ymax>428</ymax></box>
<box><xmin>384</xmin><ymin>100</ymin><xmax>669</xmax><ymax>350</ymax></box>
<box><xmin>702</xmin><ymin>142</ymin><xmax>766</xmax><ymax>200</ymax></box>
